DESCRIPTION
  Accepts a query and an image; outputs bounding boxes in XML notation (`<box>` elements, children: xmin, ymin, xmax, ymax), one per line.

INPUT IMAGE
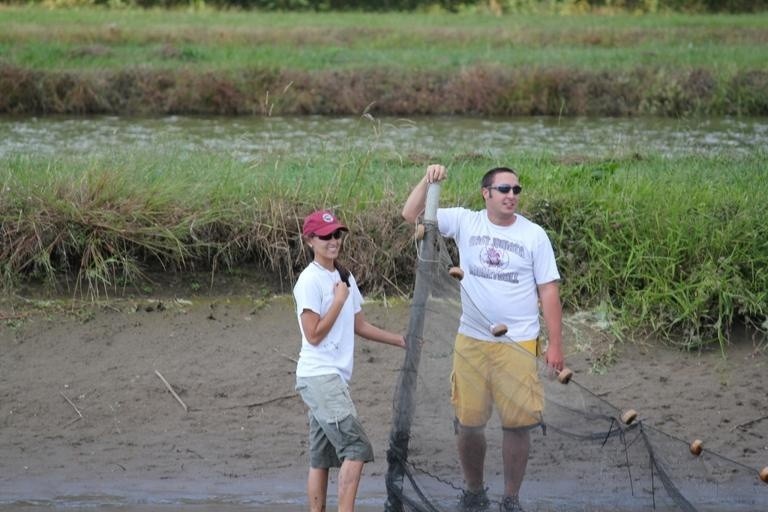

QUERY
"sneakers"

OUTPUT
<box><xmin>457</xmin><ymin>486</ymin><xmax>491</xmax><ymax>512</ymax></box>
<box><xmin>501</xmin><ymin>496</ymin><xmax>523</xmax><ymax>512</ymax></box>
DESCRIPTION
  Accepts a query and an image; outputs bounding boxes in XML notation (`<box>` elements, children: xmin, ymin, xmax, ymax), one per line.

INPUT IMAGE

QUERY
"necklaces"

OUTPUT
<box><xmin>313</xmin><ymin>263</ymin><xmax>337</xmax><ymax>285</ymax></box>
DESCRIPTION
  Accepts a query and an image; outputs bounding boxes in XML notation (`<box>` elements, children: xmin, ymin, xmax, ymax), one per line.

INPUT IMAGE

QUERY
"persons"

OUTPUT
<box><xmin>292</xmin><ymin>210</ymin><xmax>409</xmax><ymax>510</ymax></box>
<box><xmin>401</xmin><ymin>163</ymin><xmax>565</xmax><ymax>512</ymax></box>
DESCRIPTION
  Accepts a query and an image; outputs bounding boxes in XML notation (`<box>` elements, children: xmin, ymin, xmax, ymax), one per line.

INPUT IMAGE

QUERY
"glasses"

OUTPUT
<box><xmin>315</xmin><ymin>231</ymin><xmax>344</xmax><ymax>240</ymax></box>
<box><xmin>491</xmin><ymin>183</ymin><xmax>522</xmax><ymax>195</ymax></box>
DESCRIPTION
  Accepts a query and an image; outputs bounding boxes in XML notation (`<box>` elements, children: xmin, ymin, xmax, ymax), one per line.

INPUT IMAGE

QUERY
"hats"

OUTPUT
<box><xmin>303</xmin><ymin>210</ymin><xmax>349</xmax><ymax>237</ymax></box>
